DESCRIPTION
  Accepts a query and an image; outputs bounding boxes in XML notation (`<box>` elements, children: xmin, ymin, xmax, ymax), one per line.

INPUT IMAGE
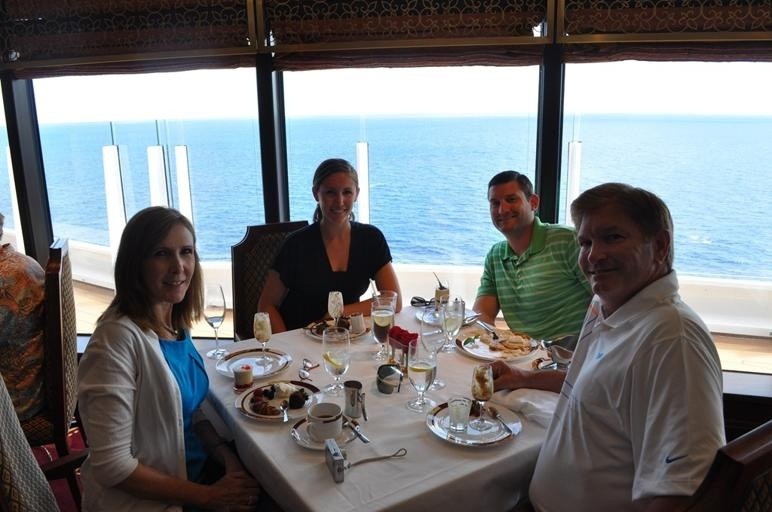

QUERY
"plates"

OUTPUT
<box><xmin>290</xmin><ymin>416</ymin><xmax>361</xmax><ymax>450</ymax></box>
<box><xmin>305</xmin><ymin>317</ymin><xmax>372</xmax><ymax>342</ymax></box>
<box><xmin>426</xmin><ymin>399</ymin><xmax>523</xmax><ymax>448</ymax></box>
<box><xmin>216</xmin><ymin>348</ymin><xmax>293</xmax><ymax>379</ymax></box>
<box><xmin>455</xmin><ymin>331</ymin><xmax>539</xmax><ymax>362</ymax></box>
<box><xmin>415</xmin><ymin>305</ymin><xmax>479</xmax><ymax>326</ymax></box>
<box><xmin>235</xmin><ymin>379</ymin><xmax>322</xmax><ymax>423</ymax></box>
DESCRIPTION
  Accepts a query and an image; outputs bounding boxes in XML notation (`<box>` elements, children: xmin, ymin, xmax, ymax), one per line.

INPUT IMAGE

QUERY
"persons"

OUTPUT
<box><xmin>471</xmin><ymin>170</ymin><xmax>593</xmax><ymax>364</ymax></box>
<box><xmin>0</xmin><ymin>215</ymin><xmax>46</xmax><ymax>426</ymax></box>
<box><xmin>74</xmin><ymin>206</ymin><xmax>278</xmax><ymax>511</ymax></box>
<box><xmin>257</xmin><ymin>159</ymin><xmax>402</xmax><ymax>335</ymax></box>
<box><xmin>486</xmin><ymin>183</ymin><xmax>727</xmax><ymax>511</ymax></box>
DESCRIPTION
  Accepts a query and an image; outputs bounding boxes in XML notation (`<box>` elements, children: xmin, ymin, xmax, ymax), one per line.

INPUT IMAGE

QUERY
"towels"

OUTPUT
<box><xmin>488</xmin><ymin>386</ymin><xmax>558</xmax><ymax>428</ymax></box>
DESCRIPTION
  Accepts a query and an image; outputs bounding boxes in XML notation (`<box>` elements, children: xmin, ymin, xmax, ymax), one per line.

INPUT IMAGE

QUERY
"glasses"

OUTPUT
<box><xmin>299</xmin><ymin>358</ymin><xmax>320</xmax><ymax>381</ymax></box>
<box><xmin>411</xmin><ymin>296</ymin><xmax>436</xmax><ymax>307</ymax></box>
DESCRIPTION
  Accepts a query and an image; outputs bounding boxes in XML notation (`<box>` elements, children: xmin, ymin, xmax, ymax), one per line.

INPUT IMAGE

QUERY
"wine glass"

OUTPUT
<box><xmin>372</xmin><ymin>290</ymin><xmax>398</xmax><ymax>315</ymax></box>
<box><xmin>469</xmin><ymin>364</ymin><xmax>494</xmax><ymax>431</ymax></box>
<box><xmin>406</xmin><ymin>338</ymin><xmax>437</xmax><ymax>413</ymax></box>
<box><xmin>371</xmin><ymin>300</ymin><xmax>395</xmax><ymax>361</ymax></box>
<box><xmin>327</xmin><ymin>291</ymin><xmax>344</xmax><ymax>327</ymax></box>
<box><xmin>439</xmin><ymin>294</ymin><xmax>463</xmax><ymax>354</ymax></box>
<box><xmin>200</xmin><ymin>284</ymin><xmax>231</xmax><ymax>360</ymax></box>
<box><xmin>253</xmin><ymin>312</ymin><xmax>273</xmax><ymax>366</ymax></box>
<box><xmin>420</xmin><ymin>306</ymin><xmax>447</xmax><ymax>392</ymax></box>
<box><xmin>323</xmin><ymin>327</ymin><xmax>350</xmax><ymax>397</ymax></box>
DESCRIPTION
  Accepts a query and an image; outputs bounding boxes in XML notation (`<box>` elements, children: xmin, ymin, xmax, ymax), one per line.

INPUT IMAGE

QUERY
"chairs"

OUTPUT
<box><xmin>0</xmin><ymin>373</ymin><xmax>88</xmax><ymax>510</ymax></box>
<box><xmin>21</xmin><ymin>238</ymin><xmax>88</xmax><ymax>510</ymax></box>
<box><xmin>687</xmin><ymin>417</ymin><xmax>772</xmax><ymax>512</ymax></box>
<box><xmin>230</xmin><ymin>220</ymin><xmax>311</xmax><ymax>345</ymax></box>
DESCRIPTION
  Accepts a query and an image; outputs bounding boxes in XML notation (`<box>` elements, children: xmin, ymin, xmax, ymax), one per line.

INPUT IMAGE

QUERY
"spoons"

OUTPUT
<box><xmin>487</xmin><ymin>407</ymin><xmax>513</xmax><ymax>434</ymax></box>
<box><xmin>280</xmin><ymin>400</ymin><xmax>289</xmax><ymax>423</ymax></box>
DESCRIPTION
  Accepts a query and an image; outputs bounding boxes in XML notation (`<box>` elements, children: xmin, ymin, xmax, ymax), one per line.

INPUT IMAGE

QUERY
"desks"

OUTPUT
<box><xmin>196</xmin><ymin>306</ymin><xmax>571</xmax><ymax>512</ymax></box>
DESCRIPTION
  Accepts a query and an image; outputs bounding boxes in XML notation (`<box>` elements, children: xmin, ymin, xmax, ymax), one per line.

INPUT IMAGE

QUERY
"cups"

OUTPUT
<box><xmin>344</xmin><ymin>381</ymin><xmax>367</xmax><ymax>421</ymax></box>
<box><xmin>233</xmin><ymin>364</ymin><xmax>255</xmax><ymax>394</ymax></box>
<box><xmin>349</xmin><ymin>311</ymin><xmax>366</xmax><ymax>334</ymax></box>
<box><xmin>447</xmin><ymin>395</ymin><xmax>472</xmax><ymax>434</ymax></box>
<box><xmin>306</xmin><ymin>402</ymin><xmax>343</xmax><ymax>443</ymax></box>
<box><xmin>435</xmin><ymin>281</ymin><xmax>449</xmax><ymax>311</ymax></box>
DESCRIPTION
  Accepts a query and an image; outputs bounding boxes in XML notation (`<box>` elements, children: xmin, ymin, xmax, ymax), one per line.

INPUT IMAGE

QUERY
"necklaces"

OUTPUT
<box><xmin>155</xmin><ymin>318</ymin><xmax>179</xmax><ymax>337</ymax></box>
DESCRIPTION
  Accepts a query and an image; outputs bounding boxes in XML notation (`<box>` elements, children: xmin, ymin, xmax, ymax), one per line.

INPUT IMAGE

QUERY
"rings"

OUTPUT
<box><xmin>248</xmin><ymin>494</ymin><xmax>254</xmax><ymax>506</ymax></box>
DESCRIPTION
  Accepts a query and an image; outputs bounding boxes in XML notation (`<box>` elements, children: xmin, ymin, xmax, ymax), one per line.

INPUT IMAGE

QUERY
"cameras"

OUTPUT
<box><xmin>325</xmin><ymin>437</ymin><xmax>345</xmax><ymax>484</ymax></box>
